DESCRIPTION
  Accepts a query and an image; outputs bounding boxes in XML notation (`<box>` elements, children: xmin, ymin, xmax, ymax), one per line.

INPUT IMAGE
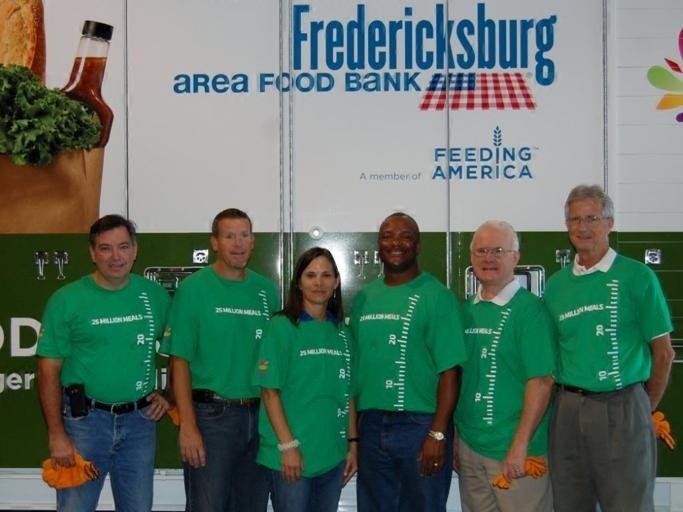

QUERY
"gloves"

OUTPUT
<box><xmin>492</xmin><ymin>457</ymin><xmax>548</xmax><ymax>489</ymax></box>
<box><xmin>42</xmin><ymin>453</ymin><xmax>98</xmax><ymax>488</ymax></box>
<box><xmin>652</xmin><ymin>410</ymin><xmax>675</xmax><ymax>451</ymax></box>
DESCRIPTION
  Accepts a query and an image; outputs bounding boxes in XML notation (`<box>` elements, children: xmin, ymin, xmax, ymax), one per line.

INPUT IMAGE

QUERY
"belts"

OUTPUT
<box><xmin>557</xmin><ymin>383</ymin><xmax>592</xmax><ymax>397</ymax></box>
<box><xmin>62</xmin><ymin>389</ymin><xmax>158</xmax><ymax>415</ymax></box>
<box><xmin>230</xmin><ymin>398</ymin><xmax>253</xmax><ymax>406</ymax></box>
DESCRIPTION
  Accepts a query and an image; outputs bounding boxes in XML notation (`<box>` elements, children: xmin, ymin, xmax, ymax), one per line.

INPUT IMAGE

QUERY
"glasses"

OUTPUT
<box><xmin>474</xmin><ymin>247</ymin><xmax>515</xmax><ymax>257</ymax></box>
<box><xmin>566</xmin><ymin>214</ymin><xmax>611</xmax><ymax>224</ymax></box>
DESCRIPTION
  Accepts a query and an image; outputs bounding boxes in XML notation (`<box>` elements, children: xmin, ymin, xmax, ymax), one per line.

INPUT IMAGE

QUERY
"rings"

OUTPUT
<box><xmin>434</xmin><ymin>463</ymin><xmax>440</xmax><ymax>469</ymax></box>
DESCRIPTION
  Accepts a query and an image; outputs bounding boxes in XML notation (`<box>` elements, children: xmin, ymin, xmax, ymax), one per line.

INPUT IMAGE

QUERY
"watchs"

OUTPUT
<box><xmin>428</xmin><ymin>430</ymin><xmax>449</xmax><ymax>442</ymax></box>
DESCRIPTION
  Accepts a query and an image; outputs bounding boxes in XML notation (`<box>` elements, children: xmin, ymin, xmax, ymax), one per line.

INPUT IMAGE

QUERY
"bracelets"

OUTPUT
<box><xmin>348</xmin><ymin>437</ymin><xmax>357</xmax><ymax>442</ymax></box>
<box><xmin>278</xmin><ymin>439</ymin><xmax>301</xmax><ymax>452</ymax></box>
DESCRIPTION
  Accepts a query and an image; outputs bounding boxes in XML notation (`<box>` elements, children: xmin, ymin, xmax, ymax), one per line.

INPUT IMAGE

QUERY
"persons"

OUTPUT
<box><xmin>249</xmin><ymin>246</ymin><xmax>359</xmax><ymax>512</ymax></box>
<box><xmin>348</xmin><ymin>212</ymin><xmax>467</xmax><ymax>512</ymax></box>
<box><xmin>453</xmin><ymin>219</ymin><xmax>558</xmax><ymax>511</ymax></box>
<box><xmin>540</xmin><ymin>183</ymin><xmax>676</xmax><ymax>512</ymax></box>
<box><xmin>33</xmin><ymin>214</ymin><xmax>173</xmax><ymax>511</ymax></box>
<box><xmin>159</xmin><ymin>208</ymin><xmax>282</xmax><ymax>512</ymax></box>
